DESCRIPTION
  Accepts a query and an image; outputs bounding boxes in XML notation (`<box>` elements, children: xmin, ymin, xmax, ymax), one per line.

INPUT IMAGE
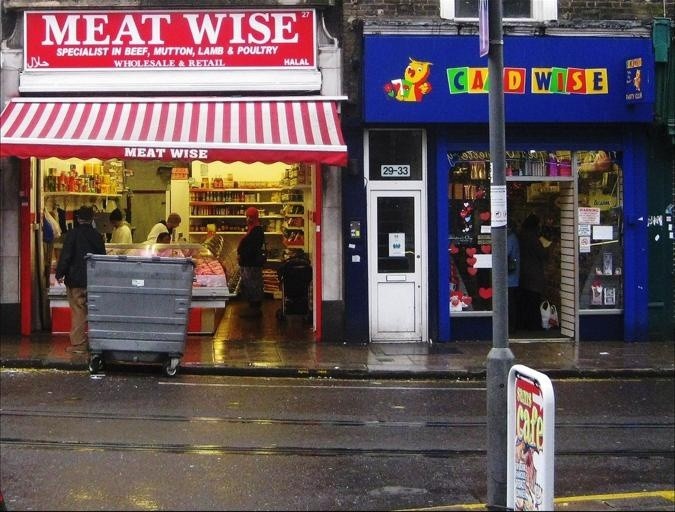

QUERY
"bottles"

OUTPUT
<box><xmin>191</xmin><ymin>191</ymin><xmax>250</xmax><ymax>232</ymax></box>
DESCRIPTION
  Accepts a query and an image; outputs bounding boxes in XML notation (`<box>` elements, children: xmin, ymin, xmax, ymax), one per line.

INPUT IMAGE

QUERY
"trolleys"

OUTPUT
<box><xmin>83</xmin><ymin>253</ymin><xmax>194</xmax><ymax>377</ymax></box>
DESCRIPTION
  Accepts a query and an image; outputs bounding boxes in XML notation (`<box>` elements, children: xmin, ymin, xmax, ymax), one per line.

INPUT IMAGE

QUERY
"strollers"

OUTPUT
<box><xmin>277</xmin><ymin>251</ymin><xmax>312</xmax><ymax>325</ymax></box>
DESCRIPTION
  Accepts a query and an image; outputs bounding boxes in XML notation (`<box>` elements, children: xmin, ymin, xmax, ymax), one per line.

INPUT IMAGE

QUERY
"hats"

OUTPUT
<box><xmin>78</xmin><ymin>205</ymin><xmax>93</xmax><ymax>219</ymax></box>
<box><xmin>109</xmin><ymin>208</ymin><xmax>122</xmax><ymax>221</ymax></box>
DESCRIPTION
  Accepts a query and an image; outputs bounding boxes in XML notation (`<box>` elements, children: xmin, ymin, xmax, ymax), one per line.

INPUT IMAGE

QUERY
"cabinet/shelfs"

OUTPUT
<box><xmin>279</xmin><ymin>188</ymin><xmax>308</xmax><ymax>253</ymax></box>
<box><xmin>46</xmin><ymin>185</ymin><xmax>121</xmax><ymax>208</ymax></box>
<box><xmin>46</xmin><ymin>244</ymin><xmax>227</xmax><ymax>336</ymax></box>
<box><xmin>187</xmin><ymin>184</ymin><xmax>281</xmax><ymax>235</ymax></box>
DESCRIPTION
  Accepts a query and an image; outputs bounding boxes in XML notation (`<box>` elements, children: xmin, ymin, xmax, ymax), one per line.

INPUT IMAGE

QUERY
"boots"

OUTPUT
<box><xmin>239</xmin><ymin>306</ymin><xmax>263</xmax><ymax>318</ymax></box>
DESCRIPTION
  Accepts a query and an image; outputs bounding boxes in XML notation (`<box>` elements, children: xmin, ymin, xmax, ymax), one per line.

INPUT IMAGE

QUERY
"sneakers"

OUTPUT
<box><xmin>65</xmin><ymin>343</ymin><xmax>86</xmax><ymax>354</ymax></box>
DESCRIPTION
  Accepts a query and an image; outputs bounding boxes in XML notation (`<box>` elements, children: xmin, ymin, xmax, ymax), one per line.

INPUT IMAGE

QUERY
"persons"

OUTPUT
<box><xmin>146</xmin><ymin>212</ymin><xmax>182</xmax><ymax>243</ymax></box>
<box><xmin>55</xmin><ymin>204</ymin><xmax>106</xmax><ymax>355</ymax></box>
<box><xmin>107</xmin><ymin>207</ymin><xmax>133</xmax><ymax>244</ymax></box>
<box><xmin>504</xmin><ymin>198</ymin><xmax>563</xmax><ymax>334</ymax></box>
<box><xmin>234</xmin><ymin>206</ymin><xmax>267</xmax><ymax>319</ymax></box>
